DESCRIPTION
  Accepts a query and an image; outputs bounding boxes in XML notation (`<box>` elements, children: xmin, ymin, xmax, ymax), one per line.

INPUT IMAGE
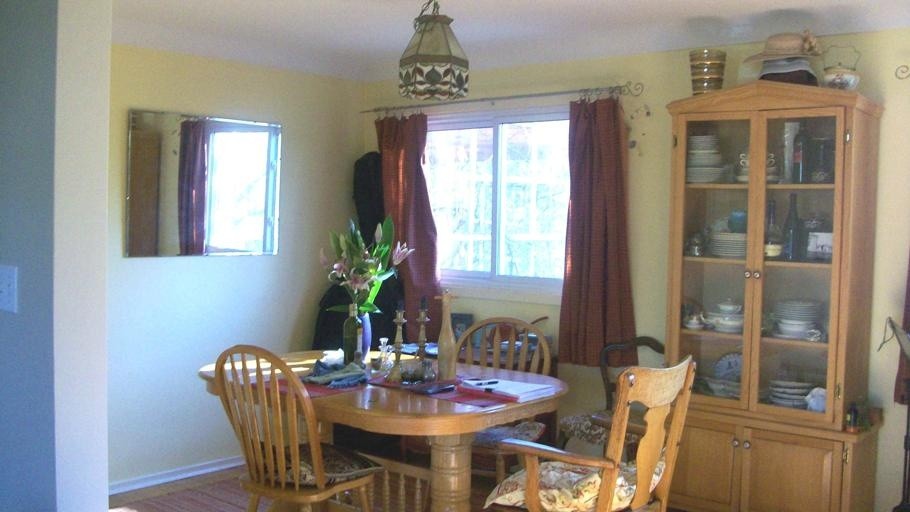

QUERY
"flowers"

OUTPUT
<box><xmin>318</xmin><ymin>213</ymin><xmax>415</xmax><ymax>314</ymax></box>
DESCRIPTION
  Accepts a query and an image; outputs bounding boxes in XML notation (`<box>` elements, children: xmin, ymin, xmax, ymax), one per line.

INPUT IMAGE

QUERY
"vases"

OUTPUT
<box><xmin>341</xmin><ymin>311</ymin><xmax>372</xmax><ymax>364</ymax></box>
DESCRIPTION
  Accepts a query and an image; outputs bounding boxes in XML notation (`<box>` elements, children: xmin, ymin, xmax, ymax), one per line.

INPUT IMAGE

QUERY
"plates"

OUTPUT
<box><xmin>683</xmin><ymin>134</ymin><xmax>821</xmax><ymax>409</ymax></box>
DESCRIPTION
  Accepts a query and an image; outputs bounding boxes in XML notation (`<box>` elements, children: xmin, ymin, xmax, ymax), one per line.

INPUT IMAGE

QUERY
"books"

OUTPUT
<box><xmin>455</xmin><ymin>378</ymin><xmax>556</xmax><ymax>403</ymax></box>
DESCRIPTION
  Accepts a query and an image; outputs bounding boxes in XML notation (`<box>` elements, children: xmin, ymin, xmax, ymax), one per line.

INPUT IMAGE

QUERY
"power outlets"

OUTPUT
<box><xmin>0</xmin><ymin>264</ymin><xmax>17</xmax><ymax>314</ymax></box>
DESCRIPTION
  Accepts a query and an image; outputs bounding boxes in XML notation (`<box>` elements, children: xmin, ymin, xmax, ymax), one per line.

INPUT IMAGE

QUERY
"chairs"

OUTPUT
<box><xmin>454</xmin><ymin>317</ymin><xmax>552</xmax><ymax>486</ymax></box>
<box><xmin>488</xmin><ymin>355</ymin><xmax>697</xmax><ymax>512</ymax></box>
<box><xmin>214</xmin><ymin>344</ymin><xmax>375</xmax><ymax>512</ymax></box>
<box><xmin>598</xmin><ymin>336</ymin><xmax>663</xmax><ymax>412</ymax></box>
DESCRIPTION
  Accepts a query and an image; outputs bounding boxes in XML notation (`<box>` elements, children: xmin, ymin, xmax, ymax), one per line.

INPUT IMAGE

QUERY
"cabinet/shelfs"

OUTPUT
<box><xmin>662</xmin><ymin>79</ymin><xmax>884</xmax><ymax>512</ymax></box>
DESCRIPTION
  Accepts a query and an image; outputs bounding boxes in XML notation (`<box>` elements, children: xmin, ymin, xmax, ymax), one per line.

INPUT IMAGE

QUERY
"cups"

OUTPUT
<box><xmin>688</xmin><ymin>48</ymin><xmax>727</xmax><ymax>95</ymax></box>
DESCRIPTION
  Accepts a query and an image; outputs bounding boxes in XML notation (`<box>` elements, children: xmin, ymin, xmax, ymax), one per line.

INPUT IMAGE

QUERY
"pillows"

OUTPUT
<box><xmin>473</xmin><ymin>421</ymin><xmax>546</xmax><ymax>449</ymax></box>
<box><xmin>559</xmin><ymin>409</ymin><xmax>647</xmax><ymax>447</ymax></box>
<box><xmin>482</xmin><ymin>442</ymin><xmax>681</xmax><ymax>512</ymax></box>
<box><xmin>246</xmin><ymin>438</ymin><xmax>384</xmax><ymax>486</ymax></box>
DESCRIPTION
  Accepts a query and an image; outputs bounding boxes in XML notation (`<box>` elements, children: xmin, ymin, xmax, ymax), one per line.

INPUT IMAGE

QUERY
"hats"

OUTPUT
<box><xmin>743</xmin><ymin>32</ymin><xmax>818</xmax><ymax>64</ymax></box>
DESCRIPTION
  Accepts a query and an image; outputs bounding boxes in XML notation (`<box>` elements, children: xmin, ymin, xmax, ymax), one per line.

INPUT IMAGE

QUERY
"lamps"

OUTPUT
<box><xmin>396</xmin><ymin>1</ymin><xmax>470</xmax><ymax>103</ymax></box>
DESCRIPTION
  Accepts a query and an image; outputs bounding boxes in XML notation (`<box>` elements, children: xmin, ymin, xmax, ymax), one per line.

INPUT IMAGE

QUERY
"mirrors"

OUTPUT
<box><xmin>124</xmin><ymin>110</ymin><xmax>283</xmax><ymax>259</ymax></box>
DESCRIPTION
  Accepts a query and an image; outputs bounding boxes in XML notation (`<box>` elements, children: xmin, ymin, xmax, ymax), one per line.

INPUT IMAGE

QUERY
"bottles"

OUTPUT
<box><xmin>342</xmin><ymin>303</ymin><xmax>364</xmax><ymax>366</ymax></box>
<box><xmin>792</xmin><ymin>119</ymin><xmax>811</xmax><ymax>182</ymax></box>
<box><xmin>846</xmin><ymin>395</ymin><xmax>871</xmax><ymax>433</ymax></box>
<box><xmin>418</xmin><ymin>359</ymin><xmax>437</xmax><ymax>382</ymax></box>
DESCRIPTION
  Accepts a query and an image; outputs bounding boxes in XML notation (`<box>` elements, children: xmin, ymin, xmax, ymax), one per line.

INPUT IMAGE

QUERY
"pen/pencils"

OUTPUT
<box><xmin>475</xmin><ymin>381</ymin><xmax>498</xmax><ymax>386</ymax></box>
